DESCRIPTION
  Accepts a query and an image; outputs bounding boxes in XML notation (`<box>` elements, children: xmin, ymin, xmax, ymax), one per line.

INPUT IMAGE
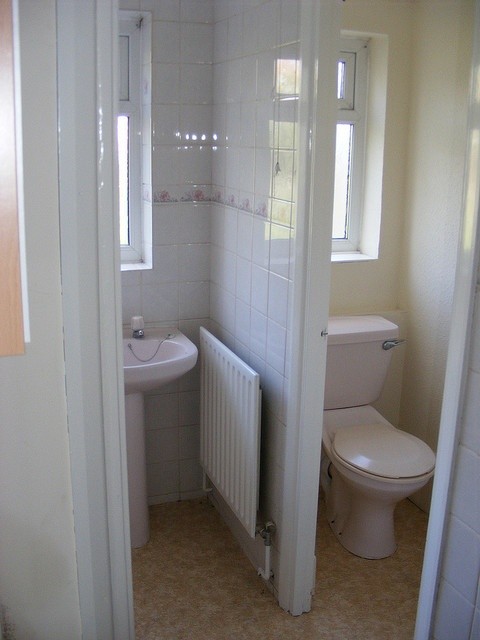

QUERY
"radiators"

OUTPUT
<box><xmin>198</xmin><ymin>325</ymin><xmax>276</xmax><ymax>583</ymax></box>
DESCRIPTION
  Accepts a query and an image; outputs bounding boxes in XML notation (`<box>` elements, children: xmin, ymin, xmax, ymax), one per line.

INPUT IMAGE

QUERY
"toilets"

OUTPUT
<box><xmin>319</xmin><ymin>315</ymin><xmax>437</xmax><ymax>561</ymax></box>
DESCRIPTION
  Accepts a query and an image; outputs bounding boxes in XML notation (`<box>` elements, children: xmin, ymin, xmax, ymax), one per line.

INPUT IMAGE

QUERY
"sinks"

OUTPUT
<box><xmin>123</xmin><ymin>325</ymin><xmax>188</xmax><ymax>368</ymax></box>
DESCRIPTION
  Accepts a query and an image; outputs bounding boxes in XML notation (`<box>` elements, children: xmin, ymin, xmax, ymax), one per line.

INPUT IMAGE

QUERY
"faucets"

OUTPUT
<box><xmin>130</xmin><ymin>315</ymin><xmax>146</xmax><ymax>339</ymax></box>
